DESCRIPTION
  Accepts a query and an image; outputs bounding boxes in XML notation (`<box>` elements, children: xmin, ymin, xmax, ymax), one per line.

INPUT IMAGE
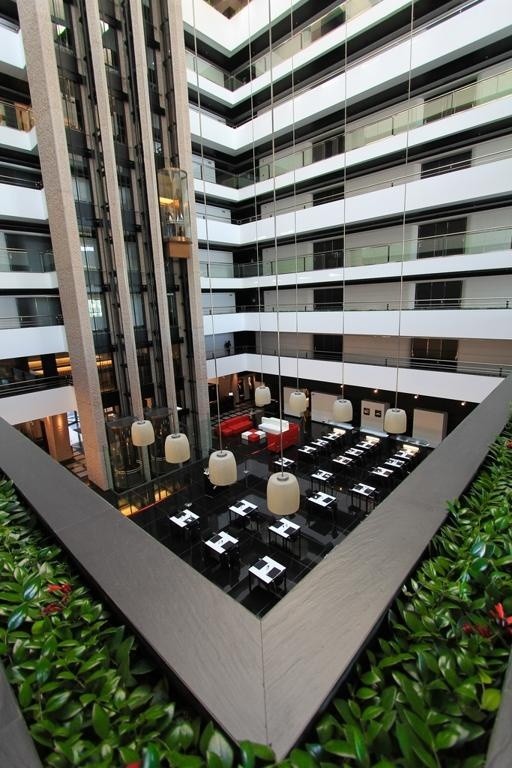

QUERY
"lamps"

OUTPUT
<box><xmin>110</xmin><ymin>79</ymin><xmax>155</xmax><ymax>446</ymax></box>
<box><xmin>267</xmin><ymin>1</ymin><xmax>300</xmax><ymax>515</ymax></box>
<box><xmin>248</xmin><ymin>0</ymin><xmax>271</xmax><ymax>406</ymax></box>
<box><xmin>332</xmin><ymin>0</ymin><xmax>353</xmax><ymax>422</ymax></box>
<box><xmin>289</xmin><ymin>0</ymin><xmax>306</xmax><ymax>415</ymax></box>
<box><xmin>192</xmin><ymin>1</ymin><xmax>238</xmax><ymax>485</ymax></box>
<box><xmin>148</xmin><ymin>0</ymin><xmax>192</xmax><ymax>463</ymax></box>
<box><xmin>384</xmin><ymin>0</ymin><xmax>413</xmax><ymax>433</ymax></box>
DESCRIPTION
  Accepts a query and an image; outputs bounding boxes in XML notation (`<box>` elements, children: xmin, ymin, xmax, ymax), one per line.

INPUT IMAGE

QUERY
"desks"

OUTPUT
<box><xmin>273</xmin><ymin>428</ymin><xmax>420</xmax><ymax>514</ymax></box>
<box><xmin>168</xmin><ymin>499</ymin><xmax>302</xmax><ymax>589</ymax></box>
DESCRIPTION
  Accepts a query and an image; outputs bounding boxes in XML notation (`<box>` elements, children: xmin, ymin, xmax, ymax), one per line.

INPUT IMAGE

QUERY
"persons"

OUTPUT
<box><xmin>165</xmin><ymin>199</ymin><xmax>184</xmax><ymax>236</ymax></box>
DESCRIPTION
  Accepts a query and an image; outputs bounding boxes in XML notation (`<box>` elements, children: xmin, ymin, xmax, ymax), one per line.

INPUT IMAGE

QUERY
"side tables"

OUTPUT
<box><xmin>241</xmin><ymin>429</ymin><xmax>266</xmax><ymax>441</ymax></box>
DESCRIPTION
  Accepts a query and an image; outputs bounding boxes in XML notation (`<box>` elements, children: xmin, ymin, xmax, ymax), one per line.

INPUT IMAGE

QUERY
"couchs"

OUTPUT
<box><xmin>258</xmin><ymin>417</ymin><xmax>300</xmax><ymax>452</ymax></box>
<box><xmin>215</xmin><ymin>414</ymin><xmax>253</xmax><ymax>437</ymax></box>
